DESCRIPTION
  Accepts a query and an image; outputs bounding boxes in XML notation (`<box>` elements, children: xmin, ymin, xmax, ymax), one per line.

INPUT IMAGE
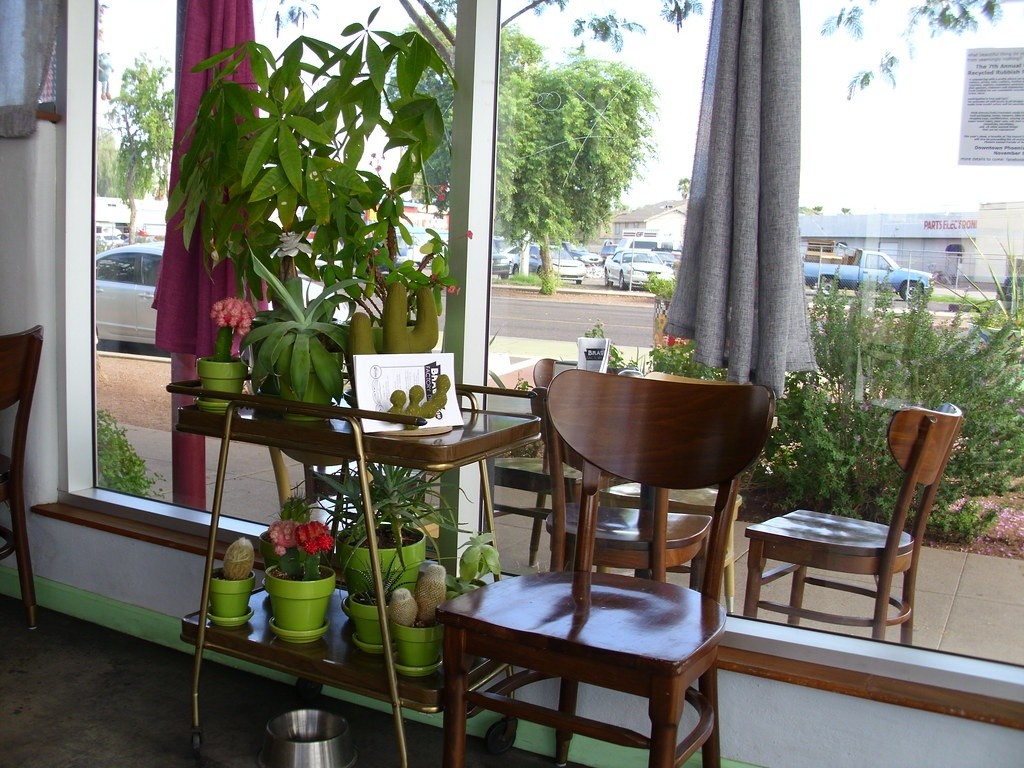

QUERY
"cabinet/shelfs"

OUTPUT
<box><xmin>165</xmin><ymin>371</ymin><xmax>566</xmax><ymax>768</ymax></box>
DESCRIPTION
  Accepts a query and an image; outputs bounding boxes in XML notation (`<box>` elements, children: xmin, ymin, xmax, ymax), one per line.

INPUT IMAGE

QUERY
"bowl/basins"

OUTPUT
<box><xmin>257</xmin><ymin>709</ymin><xmax>361</xmax><ymax>768</ymax></box>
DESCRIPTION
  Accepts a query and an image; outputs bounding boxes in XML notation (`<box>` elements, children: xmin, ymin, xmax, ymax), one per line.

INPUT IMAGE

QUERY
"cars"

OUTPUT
<box><xmin>353</xmin><ymin>223</ymin><xmax>683</xmax><ymax>292</ymax></box>
<box><xmin>89</xmin><ymin>239</ymin><xmax>353</xmax><ymax>360</ymax></box>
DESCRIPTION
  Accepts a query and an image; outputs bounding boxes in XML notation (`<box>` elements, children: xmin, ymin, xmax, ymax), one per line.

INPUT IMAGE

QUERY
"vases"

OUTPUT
<box><xmin>262</xmin><ymin>562</ymin><xmax>336</xmax><ymax>644</ymax></box>
<box><xmin>195</xmin><ymin>355</ymin><xmax>248</xmax><ymax>413</ymax></box>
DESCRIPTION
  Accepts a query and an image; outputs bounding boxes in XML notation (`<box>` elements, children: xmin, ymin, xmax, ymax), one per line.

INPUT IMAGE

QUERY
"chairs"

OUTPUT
<box><xmin>0</xmin><ymin>325</ymin><xmax>45</xmax><ymax>630</ymax></box>
<box><xmin>435</xmin><ymin>359</ymin><xmax>964</xmax><ymax>768</ymax></box>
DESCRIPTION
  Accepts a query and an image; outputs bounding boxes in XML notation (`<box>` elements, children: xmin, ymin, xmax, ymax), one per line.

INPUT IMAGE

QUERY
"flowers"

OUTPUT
<box><xmin>268</xmin><ymin>519</ymin><xmax>334</xmax><ymax>581</ymax></box>
<box><xmin>210</xmin><ymin>296</ymin><xmax>256</xmax><ymax>362</ymax></box>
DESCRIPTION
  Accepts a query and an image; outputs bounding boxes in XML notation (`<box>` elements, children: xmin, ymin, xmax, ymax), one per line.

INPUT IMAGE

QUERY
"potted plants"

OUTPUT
<box><xmin>166</xmin><ymin>4</ymin><xmax>500</xmax><ymax>679</ymax></box>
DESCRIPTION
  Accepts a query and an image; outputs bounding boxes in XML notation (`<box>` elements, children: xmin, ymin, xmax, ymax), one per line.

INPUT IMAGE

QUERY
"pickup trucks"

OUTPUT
<box><xmin>801</xmin><ymin>247</ymin><xmax>935</xmax><ymax>301</ymax></box>
